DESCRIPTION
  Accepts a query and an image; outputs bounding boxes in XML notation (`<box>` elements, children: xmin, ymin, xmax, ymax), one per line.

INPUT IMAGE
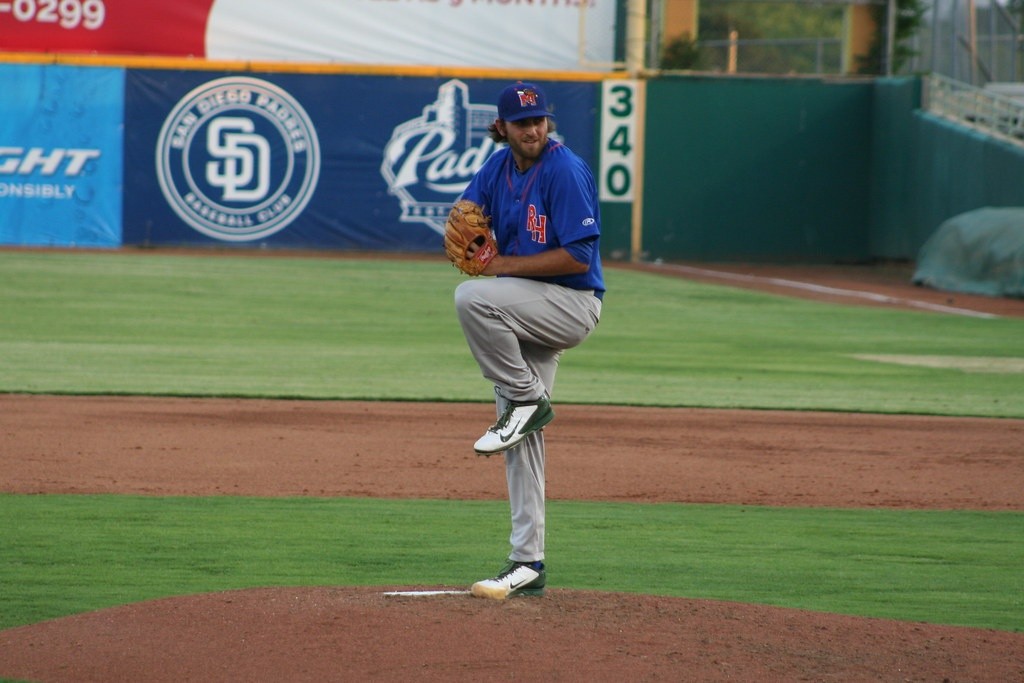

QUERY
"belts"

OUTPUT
<box><xmin>593</xmin><ymin>290</ymin><xmax>603</xmax><ymax>300</ymax></box>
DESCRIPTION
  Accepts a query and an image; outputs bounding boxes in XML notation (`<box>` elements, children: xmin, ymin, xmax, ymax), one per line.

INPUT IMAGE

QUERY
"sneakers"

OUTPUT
<box><xmin>474</xmin><ymin>386</ymin><xmax>556</xmax><ymax>458</ymax></box>
<box><xmin>471</xmin><ymin>558</ymin><xmax>546</xmax><ymax>600</ymax></box>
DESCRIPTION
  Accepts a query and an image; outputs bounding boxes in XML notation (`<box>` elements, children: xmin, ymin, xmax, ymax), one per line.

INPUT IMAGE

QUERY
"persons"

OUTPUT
<box><xmin>444</xmin><ymin>81</ymin><xmax>605</xmax><ymax>600</ymax></box>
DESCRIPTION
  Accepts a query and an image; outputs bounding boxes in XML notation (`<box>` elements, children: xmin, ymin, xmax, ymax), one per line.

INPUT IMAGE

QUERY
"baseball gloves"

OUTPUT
<box><xmin>443</xmin><ymin>199</ymin><xmax>500</xmax><ymax>278</ymax></box>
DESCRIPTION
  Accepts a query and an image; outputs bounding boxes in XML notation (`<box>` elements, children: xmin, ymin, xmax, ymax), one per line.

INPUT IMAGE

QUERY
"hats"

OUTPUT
<box><xmin>497</xmin><ymin>80</ymin><xmax>556</xmax><ymax>123</ymax></box>
